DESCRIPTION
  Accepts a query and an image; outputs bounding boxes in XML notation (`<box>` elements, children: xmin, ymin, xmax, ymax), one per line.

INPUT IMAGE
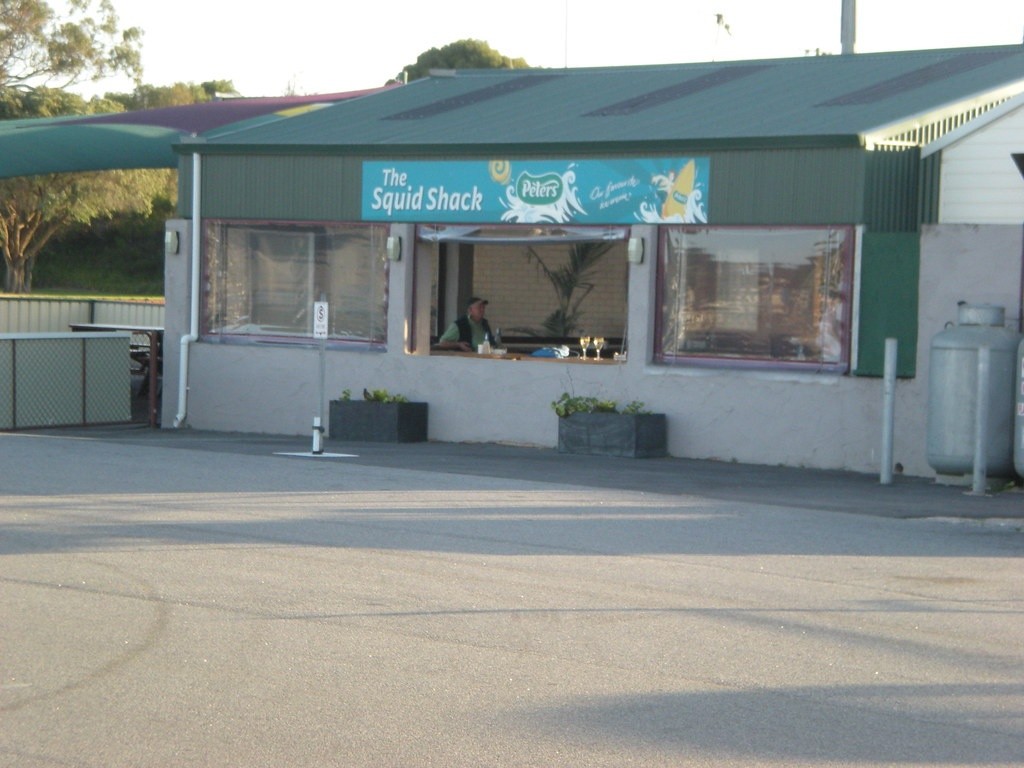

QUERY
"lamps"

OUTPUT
<box><xmin>628</xmin><ymin>237</ymin><xmax>644</xmax><ymax>263</ymax></box>
<box><xmin>386</xmin><ymin>235</ymin><xmax>400</xmax><ymax>260</ymax></box>
<box><xmin>165</xmin><ymin>230</ymin><xmax>178</xmax><ymax>254</ymax></box>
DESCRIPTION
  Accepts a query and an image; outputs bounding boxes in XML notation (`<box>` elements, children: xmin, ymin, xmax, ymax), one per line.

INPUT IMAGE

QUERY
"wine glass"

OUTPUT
<box><xmin>580</xmin><ymin>336</ymin><xmax>590</xmax><ymax>360</ymax></box>
<box><xmin>593</xmin><ymin>336</ymin><xmax>604</xmax><ymax>360</ymax></box>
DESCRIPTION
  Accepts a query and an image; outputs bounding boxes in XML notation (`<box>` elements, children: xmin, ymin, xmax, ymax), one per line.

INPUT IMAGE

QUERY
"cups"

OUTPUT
<box><xmin>478</xmin><ymin>344</ymin><xmax>483</xmax><ymax>353</ymax></box>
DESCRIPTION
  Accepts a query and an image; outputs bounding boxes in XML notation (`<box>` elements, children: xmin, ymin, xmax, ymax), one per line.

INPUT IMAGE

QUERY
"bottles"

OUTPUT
<box><xmin>661</xmin><ymin>158</ymin><xmax>696</xmax><ymax>221</ymax></box>
<box><xmin>483</xmin><ymin>333</ymin><xmax>490</xmax><ymax>354</ymax></box>
<box><xmin>495</xmin><ymin>328</ymin><xmax>502</xmax><ymax>348</ymax></box>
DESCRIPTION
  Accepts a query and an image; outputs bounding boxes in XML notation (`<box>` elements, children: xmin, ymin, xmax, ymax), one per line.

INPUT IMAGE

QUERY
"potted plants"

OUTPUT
<box><xmin>329</xmin><ymin>388</ymin><xmax>428</xmax><ymax>443</ymax></box>
<box><xmin>552</xmin><ymin>392</ymin><xmax>667</xmax><ymax>458</ymax></box>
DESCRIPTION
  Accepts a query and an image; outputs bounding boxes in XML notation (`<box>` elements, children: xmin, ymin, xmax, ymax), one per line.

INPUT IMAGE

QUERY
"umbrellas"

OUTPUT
<box><xmin>0</xmin><ymin>77</ymin><xmax>409</xmax><ymax>175</ymax></box>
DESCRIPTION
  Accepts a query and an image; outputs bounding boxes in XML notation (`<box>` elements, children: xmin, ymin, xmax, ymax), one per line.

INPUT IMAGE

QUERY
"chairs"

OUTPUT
<box><xmin>129</xmin><ymin>342</ymin><xmax>163</xmax><ymax>402</ymax></box>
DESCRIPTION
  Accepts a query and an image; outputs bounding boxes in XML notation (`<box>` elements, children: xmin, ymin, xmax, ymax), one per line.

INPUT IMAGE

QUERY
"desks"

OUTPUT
<box><xmin>68</xmin><ymin>323</ymin><xmax>164</xmax><ymax>427</ymax></box>
<box><xmin>493</xmin><ymin>342</ymin><xmax>626</xmax><ymax>350</ymax></box>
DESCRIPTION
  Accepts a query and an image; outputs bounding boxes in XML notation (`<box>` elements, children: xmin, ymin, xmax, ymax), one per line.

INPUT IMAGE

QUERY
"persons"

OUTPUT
<box><xmin>438</xmin><ymin>298</ymin><xmax>497</xmax><ymax>353</ymax></box>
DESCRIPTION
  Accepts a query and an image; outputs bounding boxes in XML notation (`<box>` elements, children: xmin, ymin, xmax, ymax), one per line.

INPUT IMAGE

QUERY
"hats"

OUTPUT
<box><xmin>466</xmin><ymin>296</ymin><xmax>488</xmax><ymax>305</ymax></box>
